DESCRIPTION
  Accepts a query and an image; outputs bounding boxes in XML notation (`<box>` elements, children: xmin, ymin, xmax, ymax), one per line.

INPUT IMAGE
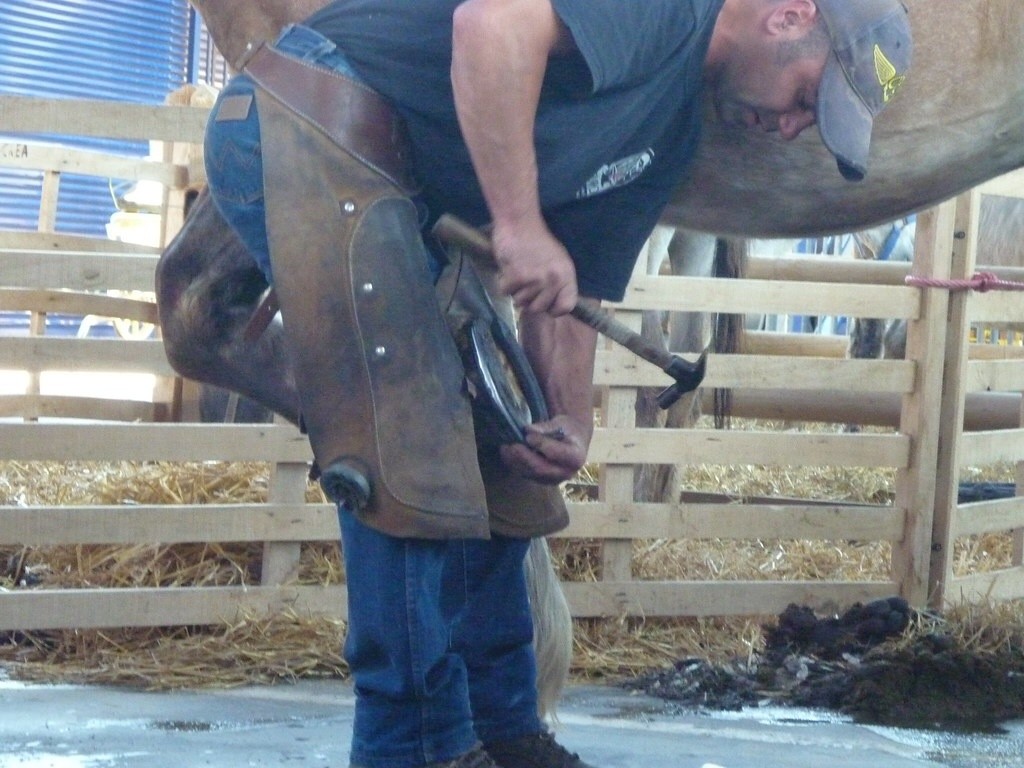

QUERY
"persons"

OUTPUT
<box><xmin>205</xmin><ymin>0</ymin><xmax>913</xmax><ymax>768</ymax></box>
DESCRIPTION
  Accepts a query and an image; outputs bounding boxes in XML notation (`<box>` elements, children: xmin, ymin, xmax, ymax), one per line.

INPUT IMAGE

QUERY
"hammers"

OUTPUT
<box><xmin>432</xmin><ymin>213</ymin><xmax>711</xmax><ymax>413</ymax></box>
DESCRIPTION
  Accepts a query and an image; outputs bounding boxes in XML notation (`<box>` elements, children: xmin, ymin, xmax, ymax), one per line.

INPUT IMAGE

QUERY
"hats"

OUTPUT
<box><xmin>814</xmin><ymin>0</ymin><xmax>913</xmax><ymax>182</ymax></box>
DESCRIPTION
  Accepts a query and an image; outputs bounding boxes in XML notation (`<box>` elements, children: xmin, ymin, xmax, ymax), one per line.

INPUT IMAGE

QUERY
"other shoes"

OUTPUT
<box><xmin>481</xmin><ymin>733</ymin><xmax>592</xmax><ymax>768</ymax></box>
<box><xmin>426</xmin><ymin>739</ymin><xmax>498</xmax><ymax>768</ymax></box>
